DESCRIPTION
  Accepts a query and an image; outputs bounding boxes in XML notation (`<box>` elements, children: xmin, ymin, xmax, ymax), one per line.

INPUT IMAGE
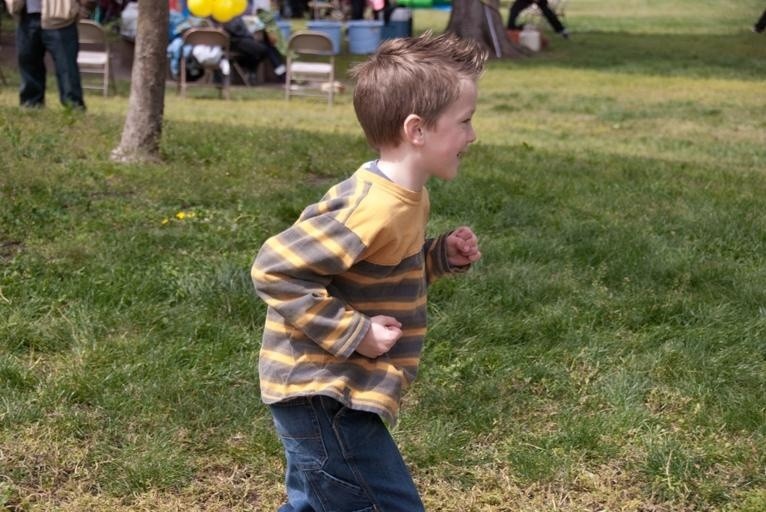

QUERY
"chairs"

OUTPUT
<box><xmin>76</xmin><ymin>20</ymin><xmax>345</xmax><ymax>102</ymax></box>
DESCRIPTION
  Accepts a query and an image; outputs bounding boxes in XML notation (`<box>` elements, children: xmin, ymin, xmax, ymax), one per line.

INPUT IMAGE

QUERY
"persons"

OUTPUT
<box><xmin>5</xmin><ymin>0</ymin><xmax>90</xmax><ymax>110</ymax></box>
<box><xmin>122</xmin><ymin>0</ymin><xmax>283</xmax><ymax>73</ymax></box>
<box><xmin>506</xmin><ymin>0</ymin><xmax>570</xmax><ymax>39</ymax></box>
<box><xmin>252</xmin><ymin>28</ymin><xmax>489</xmax><ymax>512</ymax></box>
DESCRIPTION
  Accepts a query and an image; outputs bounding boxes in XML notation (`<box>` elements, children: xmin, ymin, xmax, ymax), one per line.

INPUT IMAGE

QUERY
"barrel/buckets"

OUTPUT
<box><xmin>307</xmin><ymin>23</ymin><xmax>340</xmax><ymax>56</ymax></box>
<box><xmin>278</xmin><ymin>23</ymin><xmax>290</xmax><ymax>41</ymax></box>
<box><xmin>383</xmin><ymin>18</ymin><xmax>412</xmax><ymax>41</ymax></box>
<box><xmin>346</xmin><ymin>21</ymin><xmax>385</xmax><ymax>55</ymax></box>
